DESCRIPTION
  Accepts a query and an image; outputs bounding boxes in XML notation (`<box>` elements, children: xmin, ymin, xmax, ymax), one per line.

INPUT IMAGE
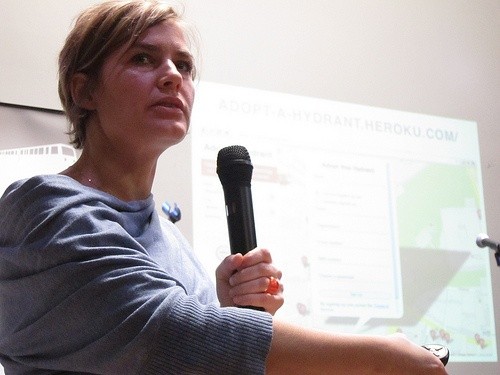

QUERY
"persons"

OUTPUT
<box><xmin>0</xmin><ymin>0</ymin><xmax>447</xmax><ymax>375</ymax></box>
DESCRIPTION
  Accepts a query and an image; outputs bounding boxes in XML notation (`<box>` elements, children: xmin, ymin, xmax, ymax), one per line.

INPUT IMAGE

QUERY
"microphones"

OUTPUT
<box><xmin>476</xmin><ymin>233</ymin><xmax>500</xmax><ymax>252</ymax></box>
<box><xmin>216</xmin><ymin>145</ymin><xmax>265</xmax><ymax>313</ymax></box>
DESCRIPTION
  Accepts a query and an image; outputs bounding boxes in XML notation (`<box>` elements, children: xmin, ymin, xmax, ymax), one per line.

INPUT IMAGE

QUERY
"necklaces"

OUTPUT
<box><xmin>78</xmin><ymin>169</ymin><xmax>107</xmax><ymax>191</ymax></box>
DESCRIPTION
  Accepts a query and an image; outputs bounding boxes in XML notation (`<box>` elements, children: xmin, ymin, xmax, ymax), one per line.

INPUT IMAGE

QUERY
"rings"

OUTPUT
<box><xmin>267</xmin><ymin>277</ymin><xmax>279</xmax><ymax>294</ymax></box>
